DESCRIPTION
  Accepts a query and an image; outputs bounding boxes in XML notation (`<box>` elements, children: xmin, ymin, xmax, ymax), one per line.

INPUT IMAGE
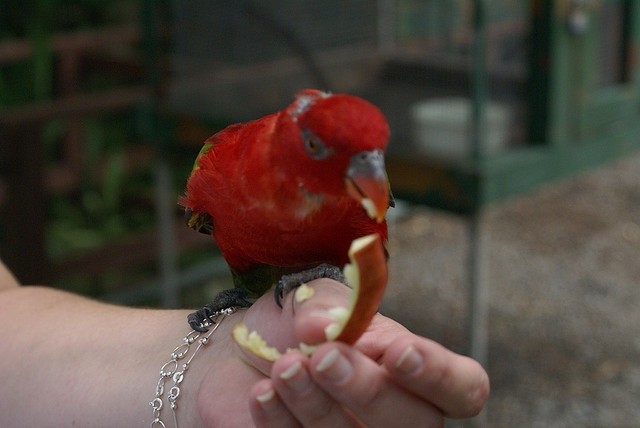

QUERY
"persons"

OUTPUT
<box><xmin>2</xmin><ymin>258</ymin><xmax>491</xmax><ymax>427</ymax></box>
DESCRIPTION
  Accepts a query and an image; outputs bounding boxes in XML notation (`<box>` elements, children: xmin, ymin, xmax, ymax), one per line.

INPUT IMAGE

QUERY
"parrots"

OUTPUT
<box><xmin>175</xmin><ymin>88</ymin><xmax>396</xmax><ymax>333</ymax></box>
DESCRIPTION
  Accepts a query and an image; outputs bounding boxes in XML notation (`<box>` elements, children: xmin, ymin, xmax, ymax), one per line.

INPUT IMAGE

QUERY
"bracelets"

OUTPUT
<box><xmin>148</xmin><ymin>302</ymin><xmax>247</xmax><ymax>427</ymax></box>
